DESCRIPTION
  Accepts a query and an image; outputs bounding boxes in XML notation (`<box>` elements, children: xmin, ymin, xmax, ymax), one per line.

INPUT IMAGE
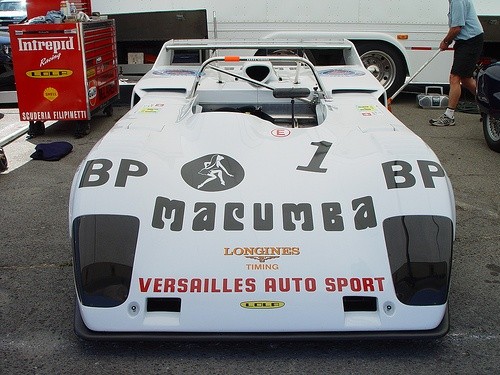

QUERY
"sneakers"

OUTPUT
<box><xmin>428</xmin><ymin>114</ymin><xmax>456</xmax><ymax>127</ymax></box>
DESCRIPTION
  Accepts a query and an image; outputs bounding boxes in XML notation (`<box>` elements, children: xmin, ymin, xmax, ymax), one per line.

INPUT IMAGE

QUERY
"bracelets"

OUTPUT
<box><xmin>443</xmin><ymin>39</ymin><xmax>452</xmax><ymax>45</ymax></box>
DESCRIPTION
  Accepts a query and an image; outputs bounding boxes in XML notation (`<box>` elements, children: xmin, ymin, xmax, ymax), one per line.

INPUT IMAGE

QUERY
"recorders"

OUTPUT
<box><xmin>417</xmin><ymin>86</ymin><xmax>449</xmax><ymax>108</ymax></box>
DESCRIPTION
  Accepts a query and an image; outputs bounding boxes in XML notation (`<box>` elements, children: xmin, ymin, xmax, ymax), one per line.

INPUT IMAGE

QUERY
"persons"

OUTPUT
<box><xmin>429</xmin><ymin>0</ymin><xmax>485</xmax><ymax>126</ymax></box>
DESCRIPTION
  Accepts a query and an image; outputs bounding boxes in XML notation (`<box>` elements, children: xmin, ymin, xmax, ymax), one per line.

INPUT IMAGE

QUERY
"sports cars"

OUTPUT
<box><xmin>67</xmin><ymin>36</ymin><xmax>457</xmax><ymax>340</ymax></box>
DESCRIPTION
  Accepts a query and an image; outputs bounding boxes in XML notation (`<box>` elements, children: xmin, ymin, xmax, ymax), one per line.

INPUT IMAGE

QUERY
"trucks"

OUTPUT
<box><xmin>3</xmin><ymin>0</ymin><xmax>500</xmax><ymax>102</ymax></box>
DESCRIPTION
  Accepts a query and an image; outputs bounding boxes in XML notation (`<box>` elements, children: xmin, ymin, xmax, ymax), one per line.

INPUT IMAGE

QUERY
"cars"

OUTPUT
<box><xmin>476</xmin><ymin>61</ymin><xmax>500</xmax><ymax>154</ymax></box>
<box><xmin>0</xmin><ymin>31</ymin><xmax>16</xmax><ymax>88</ymax></box>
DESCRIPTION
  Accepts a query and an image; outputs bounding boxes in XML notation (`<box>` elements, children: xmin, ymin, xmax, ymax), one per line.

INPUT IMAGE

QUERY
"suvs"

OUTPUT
<box><xmin>0</xmin><ymin>0</ymin><xmax>30</xmax><ymax>28</ymax></box>
